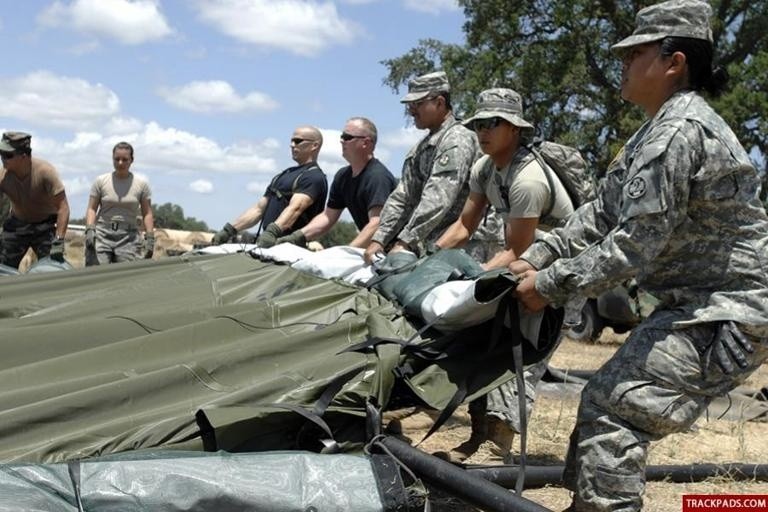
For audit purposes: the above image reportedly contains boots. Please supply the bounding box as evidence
[462,420,518,469]
[432,419,489,467]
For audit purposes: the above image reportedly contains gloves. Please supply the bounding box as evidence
[142,232,156,259]
[84,224,97,250]
[50,238,66,265]
[211,221,238,248]
[692,316,753,383]
[277,230,307,250]
[255,222,279,248]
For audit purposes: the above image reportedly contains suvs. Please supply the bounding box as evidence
[564,285,636,342]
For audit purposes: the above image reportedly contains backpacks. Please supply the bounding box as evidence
[480,141,593,227]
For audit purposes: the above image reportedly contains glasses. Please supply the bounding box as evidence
[405,92,444,112]
[289,138,316,144]
[340,133,368,143]
[473,116,507,131]
[0,152,25,160]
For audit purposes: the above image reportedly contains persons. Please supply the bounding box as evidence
[364,71,505,433]
[85,142,155,267]
[274,117,407,254]
[508,0,768,512]
[212,126,328,248]
[0,131,70,270]
[428,88,590,469]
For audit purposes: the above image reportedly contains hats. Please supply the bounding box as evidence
[0,133,31,150]
[611,0,718,52]
[460,88,536,134]
[399,70,452,100]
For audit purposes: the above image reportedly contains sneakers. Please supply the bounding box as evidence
[386,408,448,433]
[380,406,417,426]
